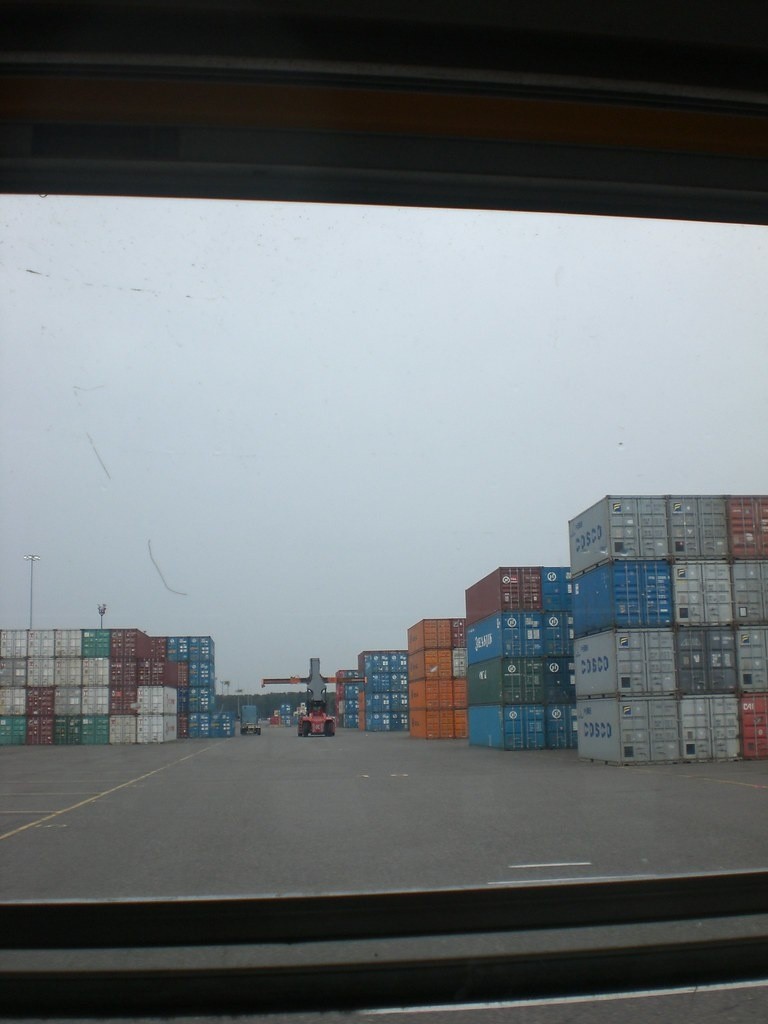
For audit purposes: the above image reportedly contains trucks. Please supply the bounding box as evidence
[240,705,262,735]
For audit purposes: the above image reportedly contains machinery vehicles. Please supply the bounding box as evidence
[263,658,368,737]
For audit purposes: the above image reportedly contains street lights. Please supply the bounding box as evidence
[23,555,40,628]
